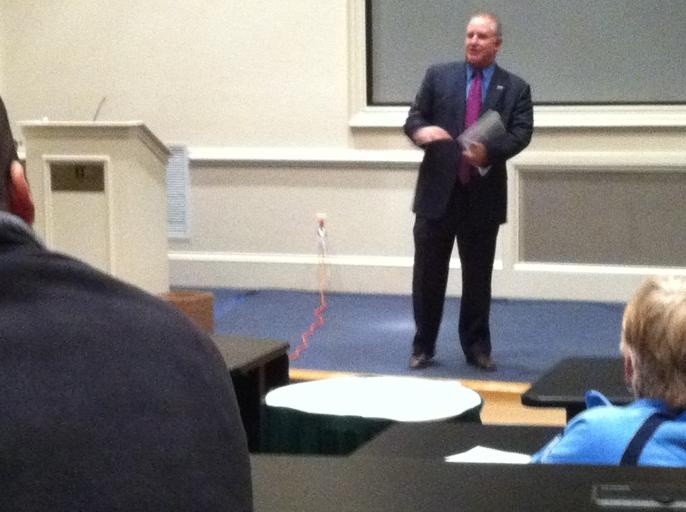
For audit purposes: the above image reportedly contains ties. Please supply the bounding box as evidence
[458,69,483,186]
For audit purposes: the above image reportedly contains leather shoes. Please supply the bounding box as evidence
[409,352,434,369]
[467,352,497,371]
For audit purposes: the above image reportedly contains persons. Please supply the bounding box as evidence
[527,272,686,470]
[402,11,533,371]
[0,97,252,511]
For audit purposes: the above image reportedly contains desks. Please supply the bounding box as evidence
[344,421,565,463]
[204,336,291,454]
[259,374,487,459]
[520,354,636,421]
